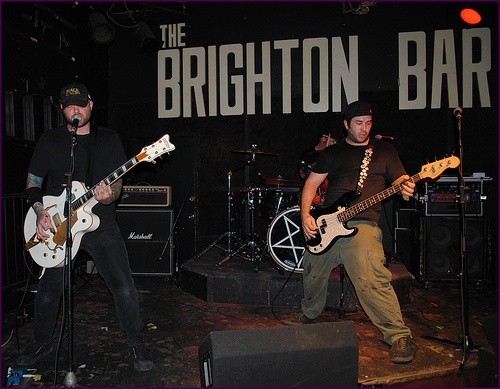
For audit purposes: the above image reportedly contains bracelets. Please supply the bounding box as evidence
[26,186,43,206]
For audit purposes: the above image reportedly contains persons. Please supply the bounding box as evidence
[303,127,343,162]
[281,100,417,362]
[16,82,154,372]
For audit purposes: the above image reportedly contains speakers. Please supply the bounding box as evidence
[116,208,174,276]
[197,320,360,388]
[417,217,490,283]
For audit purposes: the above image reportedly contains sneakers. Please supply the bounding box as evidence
[391,338,414,363]
[282,313,312,325]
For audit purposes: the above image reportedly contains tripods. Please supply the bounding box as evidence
[419,118,484,377]
[191,155,287,275]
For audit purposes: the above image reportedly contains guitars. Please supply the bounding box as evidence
[23,133,177,269]
[302,153,462,256]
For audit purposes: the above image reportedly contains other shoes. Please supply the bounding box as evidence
[130,345,154,372]
[16,338,51,366]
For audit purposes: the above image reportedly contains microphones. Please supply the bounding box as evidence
[71,114,82,132]
[453,107,462,118]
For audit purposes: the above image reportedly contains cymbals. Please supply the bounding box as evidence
[229,148,277,157]
[264,178,303,187]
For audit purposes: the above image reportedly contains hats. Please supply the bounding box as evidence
[60,82,91,107]
[344,101,373,120]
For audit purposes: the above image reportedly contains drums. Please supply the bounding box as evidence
[231,187,267,209]
[266,204,315,274]
[274,190,301,213]
